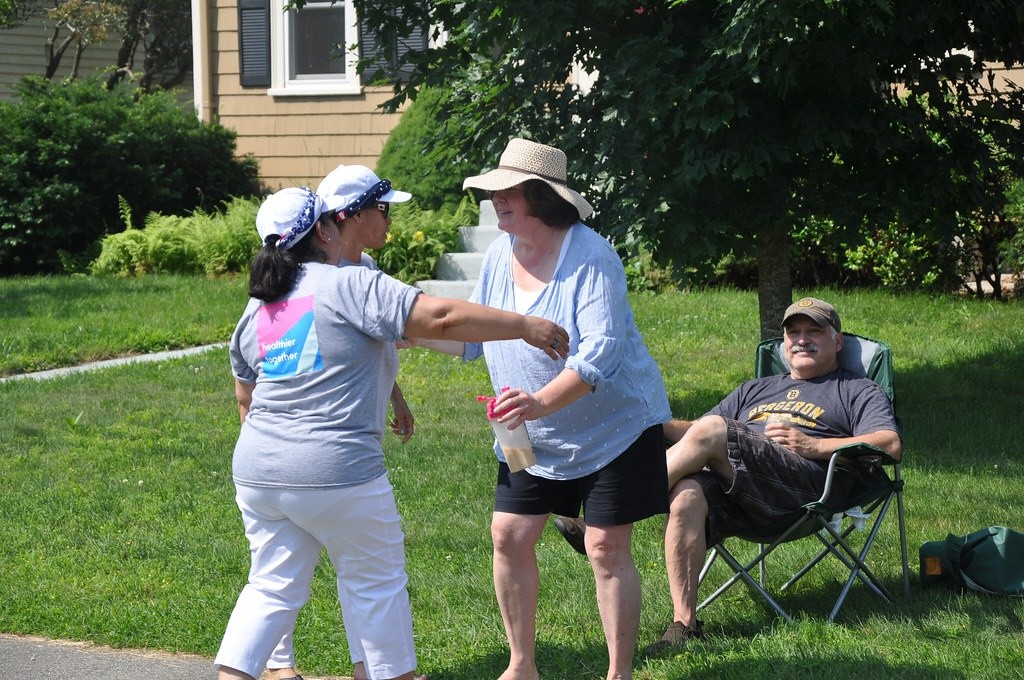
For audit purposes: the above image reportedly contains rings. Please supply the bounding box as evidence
[785,437,788,443]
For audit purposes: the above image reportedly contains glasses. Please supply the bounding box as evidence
[366,202,390,219]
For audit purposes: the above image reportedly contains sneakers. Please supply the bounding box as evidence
[552,514,588,557]
[641,620,708,658]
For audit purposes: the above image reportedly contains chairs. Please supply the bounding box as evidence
[695,332,912,626]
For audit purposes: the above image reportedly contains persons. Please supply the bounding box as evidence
[554,298,903,657]
[410,137,668,680]
[210,187,572,680]
[216,163,421,680]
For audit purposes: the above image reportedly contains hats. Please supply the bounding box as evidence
[256,186,346,250]
[461,136,595,220]
[778,297,842,334]
[316,165,413,223]
